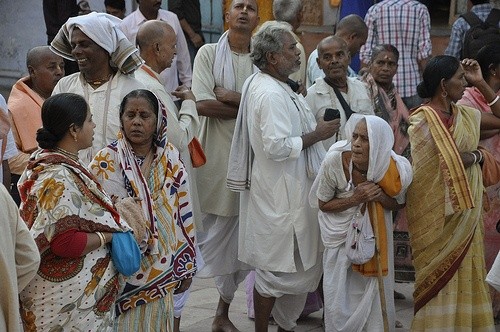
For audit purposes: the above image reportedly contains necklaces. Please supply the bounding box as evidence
[84,72,111,85]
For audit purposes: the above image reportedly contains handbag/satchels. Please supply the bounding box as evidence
[187,137,207,168]
[344,201,375,264]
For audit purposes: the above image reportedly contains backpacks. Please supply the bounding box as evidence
[460,7,500,81]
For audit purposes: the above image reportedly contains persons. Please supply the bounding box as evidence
[50,11,147,170]
[87,89,198,332]
[263,0,306,86]
[121,0,192,103]
[306,14,369,89]
[225,21,341,332]
[360,0,500,332]
[104,0,125,19]
[300,35,376,317]
[134,20,204,332]
[360,45,416,299]
[308,114,414,332]
[18,93,132,332]
[173,0,262,332]
[173,0,205,59]
[0,183,40,332]
[7,46,65,206]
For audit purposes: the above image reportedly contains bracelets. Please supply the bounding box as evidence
[487,96,499,106]
[472,150,482,163]
[96,231,106,247]
